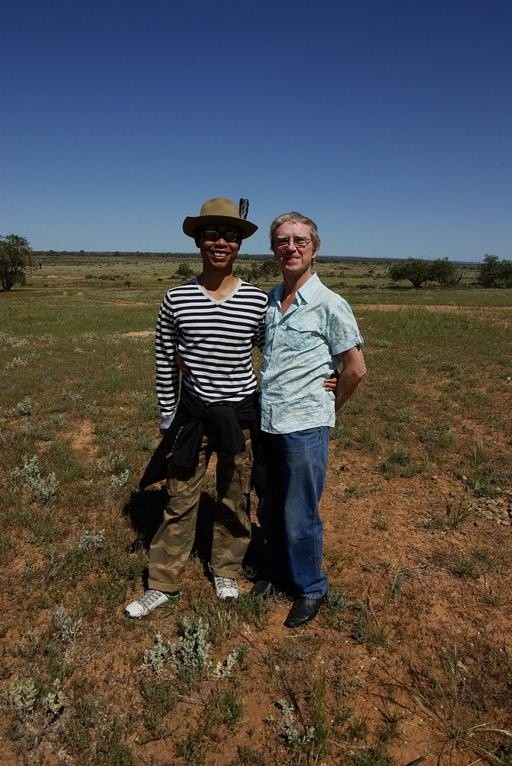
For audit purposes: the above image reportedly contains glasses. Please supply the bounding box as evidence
[199,228,242,242]
[276,236,312,247]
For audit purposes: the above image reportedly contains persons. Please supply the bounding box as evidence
[173,212,368,629]
[121,198,339,621]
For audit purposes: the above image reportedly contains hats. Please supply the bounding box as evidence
[182,198,258,239]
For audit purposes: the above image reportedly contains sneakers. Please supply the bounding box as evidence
[124,589,183,620]
[213,576,239,602]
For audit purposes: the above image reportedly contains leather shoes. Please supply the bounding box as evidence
[249,576,275,602]
[283,588,329,628]
